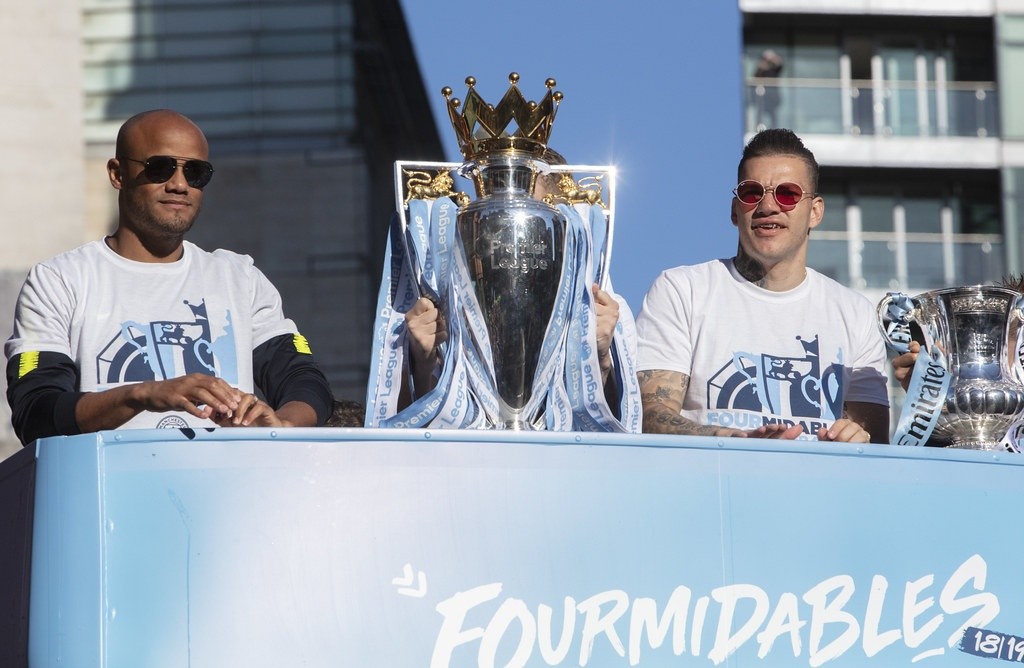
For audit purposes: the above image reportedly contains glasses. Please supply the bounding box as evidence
[118,155,216,188]
[732,179,818,206]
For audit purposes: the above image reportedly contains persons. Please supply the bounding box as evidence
[4,107,336,446]
[891,340,920,394]
[404,145,620,406]
[636,128,887,443]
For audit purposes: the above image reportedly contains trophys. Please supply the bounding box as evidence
[878,286,1023,451]
[393,73,619,431]
